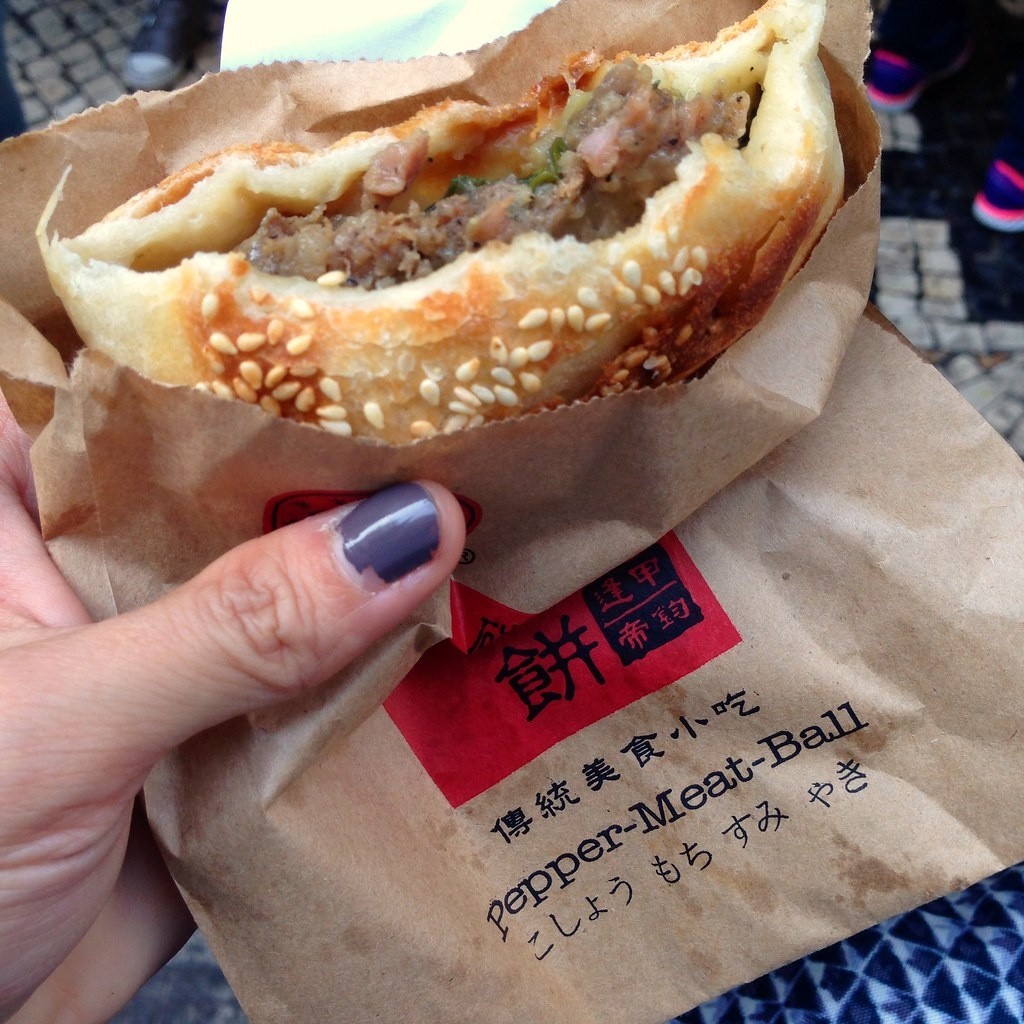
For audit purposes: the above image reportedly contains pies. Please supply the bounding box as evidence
[31,0,849,438]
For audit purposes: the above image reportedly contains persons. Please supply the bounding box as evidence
[862,0,1024,233]
[0,394,463,1024]
[121,0,207,93]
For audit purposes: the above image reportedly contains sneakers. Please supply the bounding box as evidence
[972,160,1024,232]
[863,48,919,113]
[122,0,209,91]
